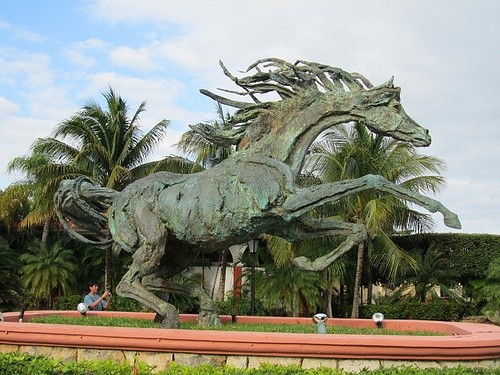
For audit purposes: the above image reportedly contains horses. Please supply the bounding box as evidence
[50,56,463,329]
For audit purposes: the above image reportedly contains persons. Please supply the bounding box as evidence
[84,279,113,311]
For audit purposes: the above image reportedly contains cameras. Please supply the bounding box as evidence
[105,292,112,298]
